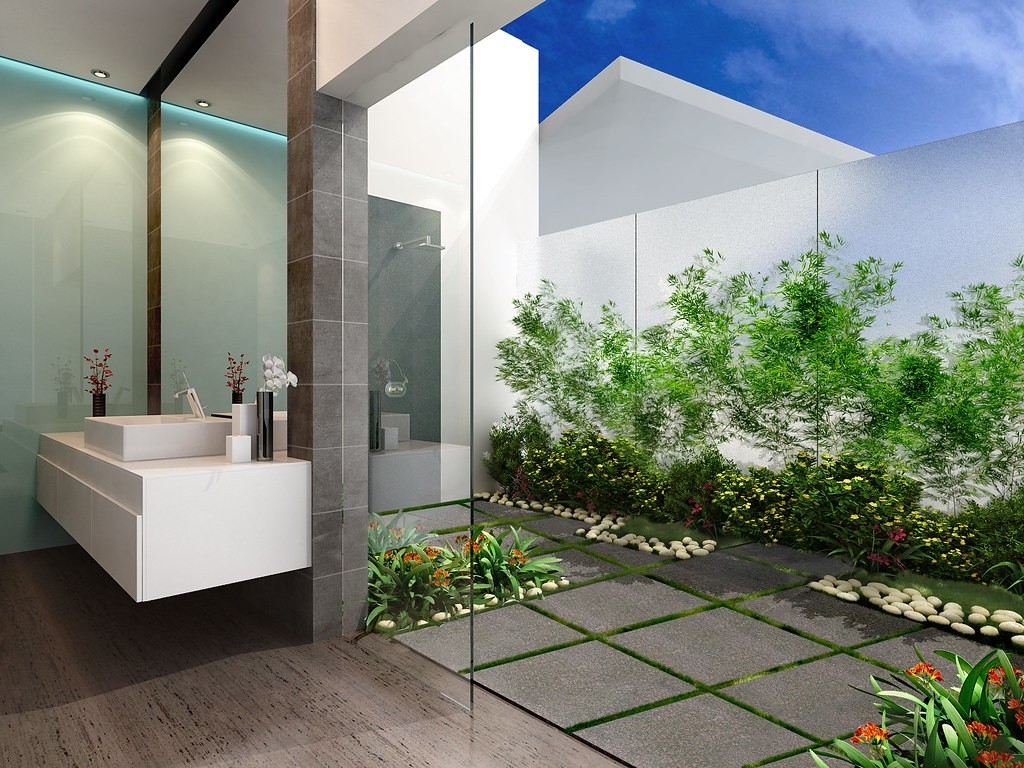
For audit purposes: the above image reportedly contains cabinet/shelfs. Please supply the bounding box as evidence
[35,452,146,603]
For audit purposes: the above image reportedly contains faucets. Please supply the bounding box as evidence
[174,388,209,417]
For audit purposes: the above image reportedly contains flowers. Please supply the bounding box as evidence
[50,356,74,393]
[368,356,392,392]
[169,358,188,397]
[224,352,250,394]
[84,347,113,394]
[256,354,298,398]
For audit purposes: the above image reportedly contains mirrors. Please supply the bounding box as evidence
[159,0,289,418]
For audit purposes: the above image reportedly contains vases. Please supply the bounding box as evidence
[231,393,243,404]
[175,398,183,414]
[92,393,106,417]
[370,389,380,453]
[58,392,69,419]
[257,392,274,463]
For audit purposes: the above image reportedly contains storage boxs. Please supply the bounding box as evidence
[380,426,400,451]
[226,435,251,463]
[232,403,257,459]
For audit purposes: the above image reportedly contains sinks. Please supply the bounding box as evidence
[380,412,411,441]
[83,413,232,463]
